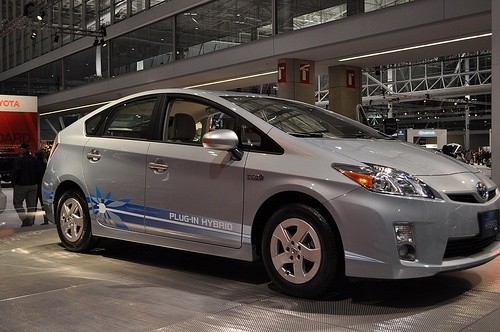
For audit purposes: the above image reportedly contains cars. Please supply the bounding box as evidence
[41,89,500,300]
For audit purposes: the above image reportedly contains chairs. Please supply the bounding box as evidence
[170,113,196,143]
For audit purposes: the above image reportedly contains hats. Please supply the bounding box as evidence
[19,144,29,150]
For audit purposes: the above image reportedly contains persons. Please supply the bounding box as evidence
[33,149,50,226]
[458,147,493,167]
[9,143,43,229]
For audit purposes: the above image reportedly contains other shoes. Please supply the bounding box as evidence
[28,224,33,226]
[40,222,49,226]
[21,218,31,227]
